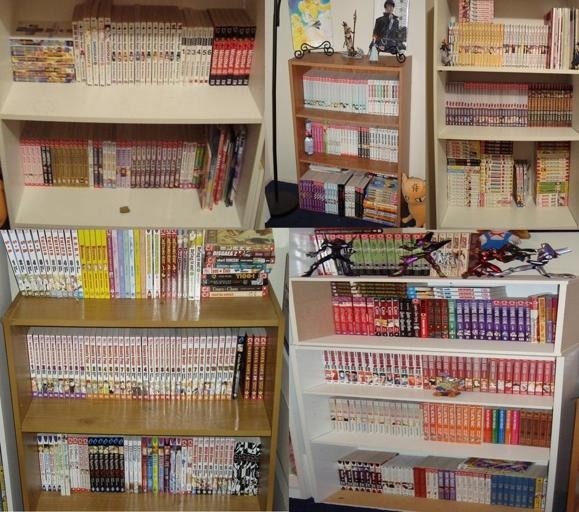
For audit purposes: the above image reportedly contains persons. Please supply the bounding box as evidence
[373,0,400,41]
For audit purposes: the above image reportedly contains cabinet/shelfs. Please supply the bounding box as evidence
[0,0,579,512]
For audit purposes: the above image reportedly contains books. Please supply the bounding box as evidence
[8,0,257,88]
[302,68,400,117]
[323,350,556,397]
[446,139,571,210]
[304,120,399,163]
[448,0,579,70]
[336,449,547,510]
[298,165,399,227]
[332,396,553,448]
[444,82,573,127]
[312,230,470,277]
[26,326,267,401]
[331,282,558,345]
[0,228,275,301]
[18,125,247,212]
[36,432,271,496]
